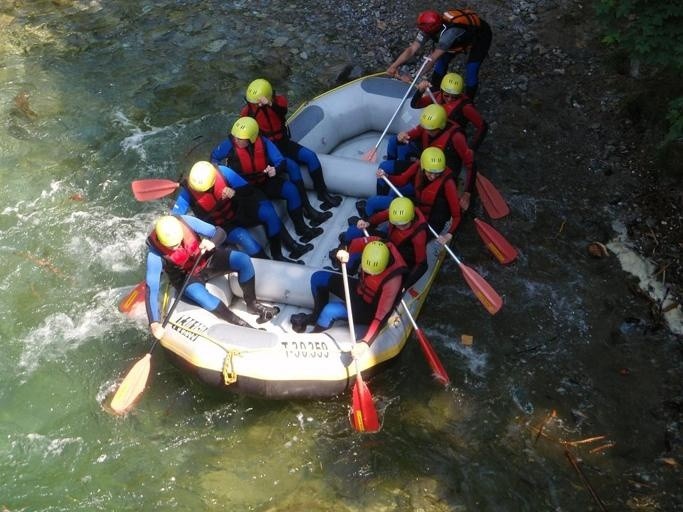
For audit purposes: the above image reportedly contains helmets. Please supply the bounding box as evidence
[420,146,447,173]
[420,103,447,131]
[388,197,417,226]
[244,77,273,106]
[229,116,260,146]
[155,215,185,249]
[361,241,391,276]
[416,9,443,35]
[440,72,465,95]
[188,160,217,193]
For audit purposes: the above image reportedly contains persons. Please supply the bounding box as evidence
[385,8,492,100]
[145,213,280,340]
[386,72,487,162]
[329,197,429,289]
[347,148,461,247]
[290,236,408,361]
[169,160,304,265]
[377,103,474,211]
[239,79,342,228]
[211,117,323,260]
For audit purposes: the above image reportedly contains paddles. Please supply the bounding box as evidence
[118,279,147,314]
[112,247,207,412]
[382,174,503,315]
[364,229,450,385]
[362,56,431,163]
[427,87,510,219]
[132,180,180,202]
[342,263,380,432]
[408,139,518,266]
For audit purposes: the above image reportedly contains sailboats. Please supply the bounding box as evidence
[142,69,477,399]
